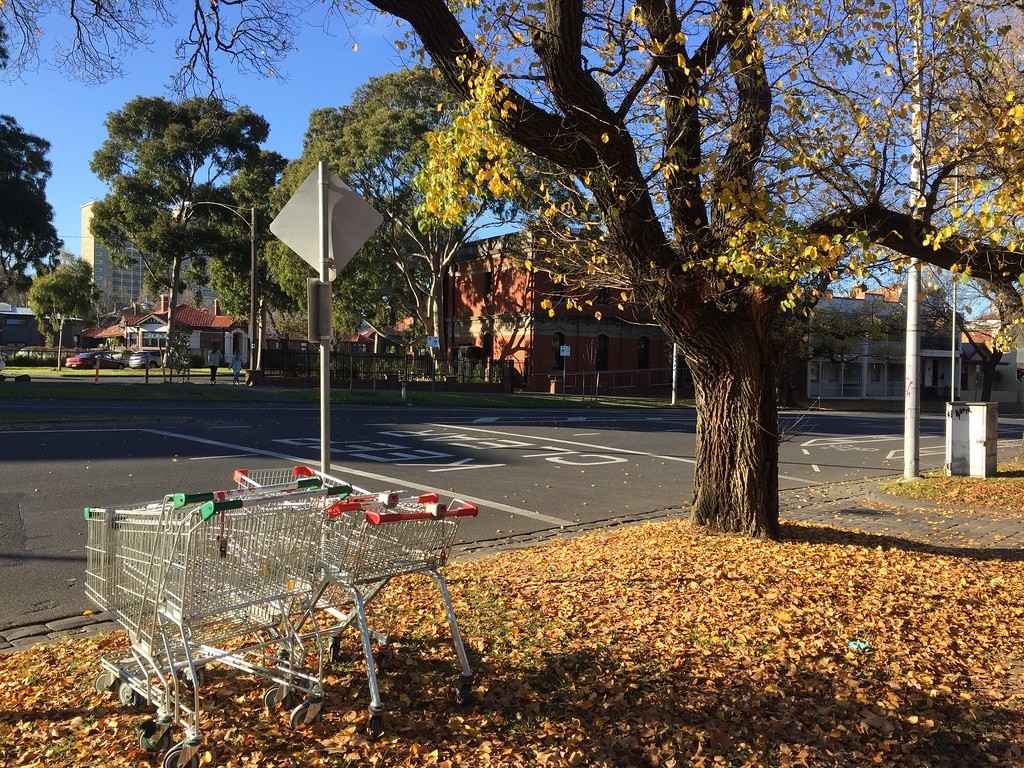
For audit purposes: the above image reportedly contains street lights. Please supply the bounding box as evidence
[182,202,266,388]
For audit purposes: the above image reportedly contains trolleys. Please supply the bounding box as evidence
[82,465,481,768]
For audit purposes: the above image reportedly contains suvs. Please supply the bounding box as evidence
[129,351,164,368]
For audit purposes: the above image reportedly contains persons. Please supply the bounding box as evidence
[208,344,223,385]
[228,348,244,385]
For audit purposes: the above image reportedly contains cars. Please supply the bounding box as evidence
[65,352,127,370]
